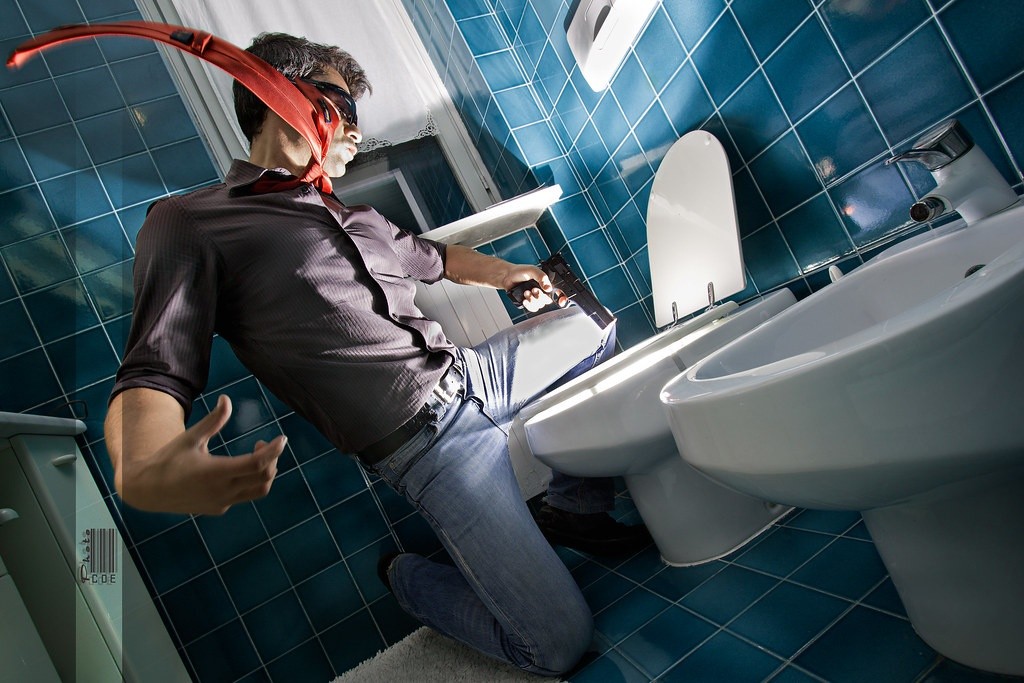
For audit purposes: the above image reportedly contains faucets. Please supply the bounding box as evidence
[881,121,1019,226]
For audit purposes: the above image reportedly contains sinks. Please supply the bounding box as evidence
[661,199,1024,675]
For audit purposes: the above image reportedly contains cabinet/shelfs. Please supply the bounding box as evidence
[0,433,193,683]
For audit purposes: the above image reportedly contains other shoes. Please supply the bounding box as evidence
[555,513,648,550]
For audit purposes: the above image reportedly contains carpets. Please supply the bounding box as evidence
[329,626,568,683]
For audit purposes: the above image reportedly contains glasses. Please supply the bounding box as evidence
[290,78,357,127]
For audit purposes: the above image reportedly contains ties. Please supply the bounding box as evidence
[8,21,342,193]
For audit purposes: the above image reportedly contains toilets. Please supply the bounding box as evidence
[521,124,798,573]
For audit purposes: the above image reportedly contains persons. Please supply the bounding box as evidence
[106,33,616,674]
